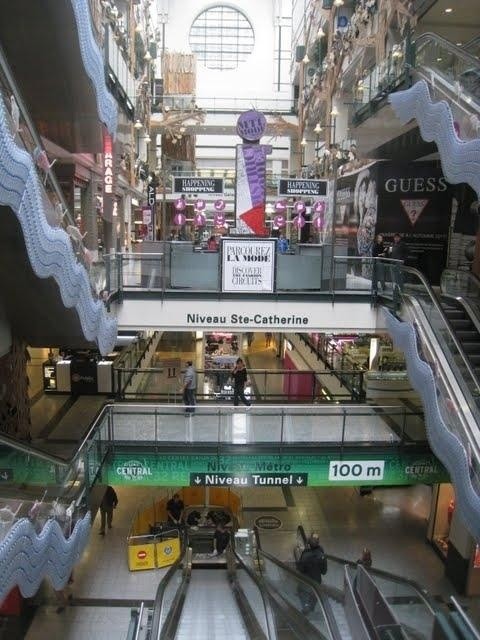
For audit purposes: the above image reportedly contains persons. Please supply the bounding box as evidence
[306,235,316,244]
[230,356,252,411]
[278,235,289,255]
[207,235,217,249]
[372,233,387,290]
[179,358,197,416]
[265,332,273,348]
[351,548,374,589]
[212,522,230,556]
[295,532,328,615]
[49,571,73,614]
[166,494,185,527]
[246,333,253,346]
[390,232,410,290]
[98,485,118,536]
